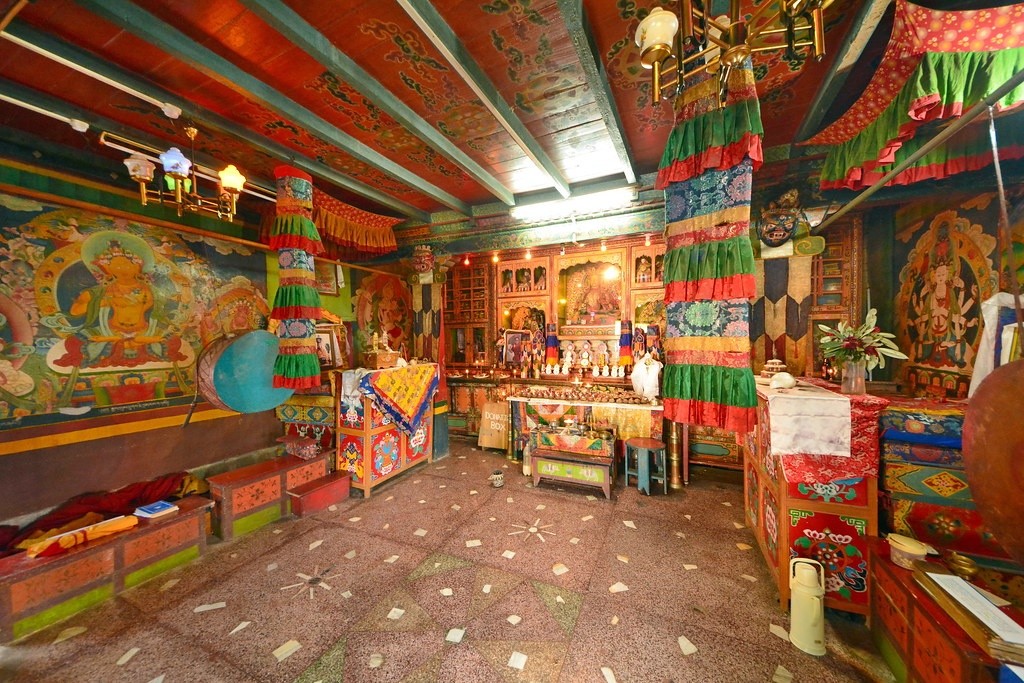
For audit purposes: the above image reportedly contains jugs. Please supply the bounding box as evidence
[789,556,827,656]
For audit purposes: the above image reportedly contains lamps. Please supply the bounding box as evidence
[123,128,246,223]
[635,0,835,110]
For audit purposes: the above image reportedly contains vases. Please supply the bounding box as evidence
[840,360,866,395]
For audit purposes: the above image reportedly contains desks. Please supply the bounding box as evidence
[506,385,664,486]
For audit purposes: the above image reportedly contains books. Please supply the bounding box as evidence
[133,500,179,519]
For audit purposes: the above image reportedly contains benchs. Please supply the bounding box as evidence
[0,494,215,647]
[205,446,338,542]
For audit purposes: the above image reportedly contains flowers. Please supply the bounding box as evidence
[817,308,909,373]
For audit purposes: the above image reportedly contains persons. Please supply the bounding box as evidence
[636,257,652,283]
[572,266,619,315]
[501,272,513,292]
[534,269,546,290]
[509,337,522,361]
[516,270,531,292]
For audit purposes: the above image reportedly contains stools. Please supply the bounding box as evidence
[287,469,352,516]
[624,437,667,495]
